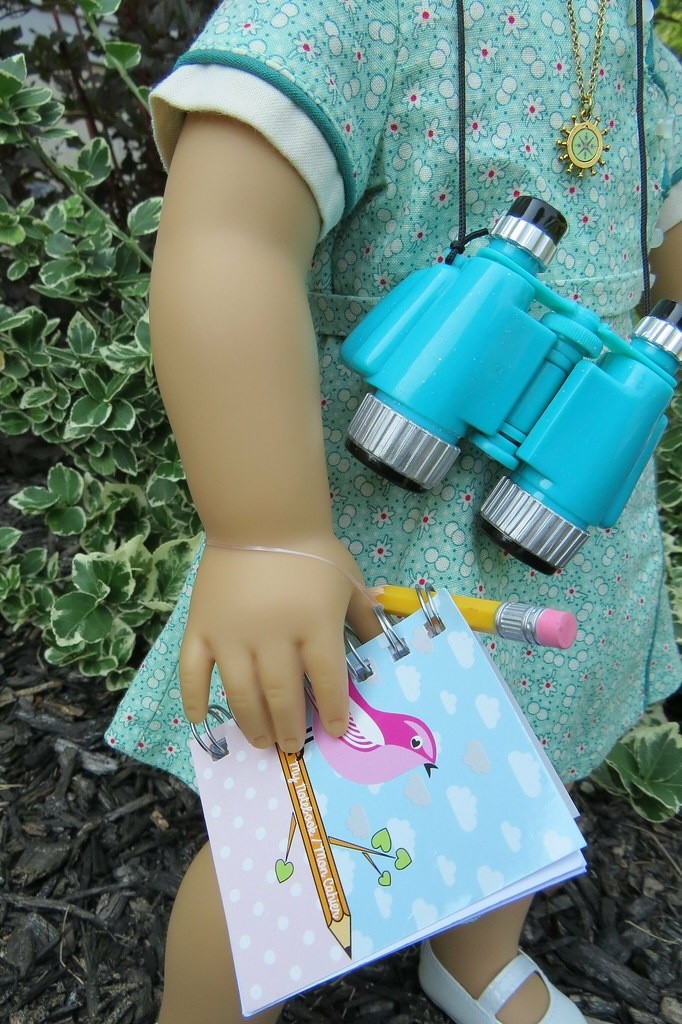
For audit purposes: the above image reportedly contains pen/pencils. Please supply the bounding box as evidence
[368,585,577,649]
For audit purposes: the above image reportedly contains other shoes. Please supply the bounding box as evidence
[419,937,590,1024]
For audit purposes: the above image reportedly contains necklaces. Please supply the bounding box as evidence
[555,0,611,179]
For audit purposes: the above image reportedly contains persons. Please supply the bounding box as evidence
[103,0,682,1024]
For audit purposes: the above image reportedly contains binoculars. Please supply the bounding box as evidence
[340,195,682,575]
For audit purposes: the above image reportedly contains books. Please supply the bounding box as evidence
[183,582,589,1016]
